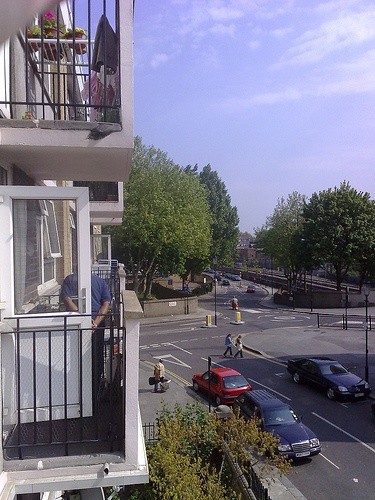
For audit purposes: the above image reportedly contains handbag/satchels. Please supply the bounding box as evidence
[149,376,156,385]
[160,378,169,392]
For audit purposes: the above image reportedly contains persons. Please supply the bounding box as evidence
[233,336,243,359]
[230,296,238,307]
[62,263,111,404]
[93,253,104,265]
[223,333,233,357]
[152,359,165,392]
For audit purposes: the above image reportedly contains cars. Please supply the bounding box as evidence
[204,268,242,281]
[247,285,256,293]
[286,356,371,403]
[222,280,230,286]
[192,368,252,408]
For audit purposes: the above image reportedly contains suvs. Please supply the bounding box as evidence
[231,389,321,462]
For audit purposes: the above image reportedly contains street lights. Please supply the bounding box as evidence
[362,283,370,383]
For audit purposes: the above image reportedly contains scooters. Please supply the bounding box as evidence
[230,301,239,310]
